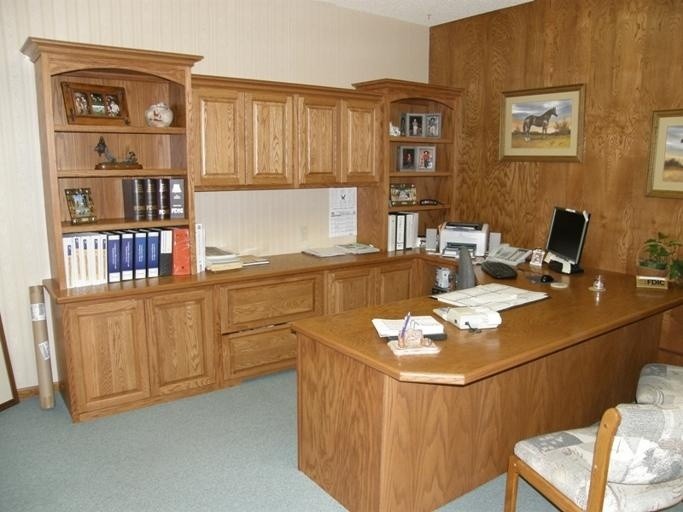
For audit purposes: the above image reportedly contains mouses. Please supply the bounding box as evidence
[541,275,553,284]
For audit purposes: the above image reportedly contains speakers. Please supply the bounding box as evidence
[425,229,437,251]
[489,233,501,252]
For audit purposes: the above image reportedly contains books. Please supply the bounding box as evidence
[431,283,550,313]
[124,177,184,219]
[64,227,191,287]
[194,225,269,272]
[388,211,418,251]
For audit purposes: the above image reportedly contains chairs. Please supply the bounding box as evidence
[502,402,683,512]
[636,359,683,410]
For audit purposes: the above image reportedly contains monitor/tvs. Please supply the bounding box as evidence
[543,206,591,275]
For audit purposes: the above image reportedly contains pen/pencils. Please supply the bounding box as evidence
[401,311,410,337]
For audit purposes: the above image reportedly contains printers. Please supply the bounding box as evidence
[439,222,489,258]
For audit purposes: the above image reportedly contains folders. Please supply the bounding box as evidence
[387,214,396,252]
[396,215,406,251]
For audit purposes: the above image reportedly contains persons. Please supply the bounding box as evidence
[406,152,411,168]
[412,117,418,135]
[428,117,436,135]
[422,152,429,168]
[73,91,119,114]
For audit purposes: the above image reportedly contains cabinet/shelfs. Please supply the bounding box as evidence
[191,76,295,193]
[19,36,203,290]
[353,79,466,253]
[42,244,420,424]
[293,84,383,188]
[422,248,473,298]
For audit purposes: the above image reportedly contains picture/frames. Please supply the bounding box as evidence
[396,145,416,171]
[416,145,436,170]
[389,185,417,206]
[62,81,130,126]
[425,113,442,139]
[406,114,425,138]
[645,108,683,199]
[399,112,406,135]
[64,187,99,223]
[500,84,585,162]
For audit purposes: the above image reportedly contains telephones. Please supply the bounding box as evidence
[486,243,532,266]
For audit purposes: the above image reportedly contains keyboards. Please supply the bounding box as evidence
[481,260,517,279]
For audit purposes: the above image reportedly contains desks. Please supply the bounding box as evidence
[288,254,683,512]
